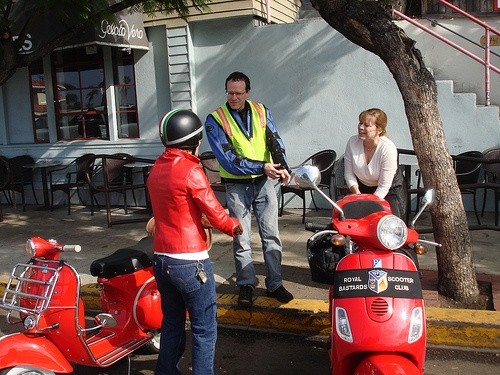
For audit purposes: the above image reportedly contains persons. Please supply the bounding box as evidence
[203,70,295,303]
[144,109,242,375]
[342,107,409,260]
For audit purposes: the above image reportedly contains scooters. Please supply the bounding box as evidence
[0,220,214,375]
[295,165,443,375]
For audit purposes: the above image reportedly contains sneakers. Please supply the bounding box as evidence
[238,284,252,306]
[267,285,293,302]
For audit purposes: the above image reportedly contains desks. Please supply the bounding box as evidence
[398,154,429,231]
[121,158,157,218]
[21,158,67,214]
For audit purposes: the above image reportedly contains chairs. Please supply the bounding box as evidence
[0,147,500,236]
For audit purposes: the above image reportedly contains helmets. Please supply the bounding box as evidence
[159,109,203,150]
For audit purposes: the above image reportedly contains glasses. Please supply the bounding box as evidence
[225,90,247,98]
